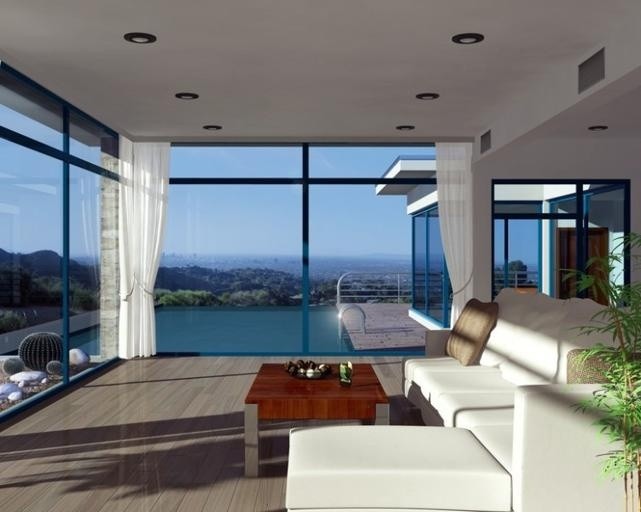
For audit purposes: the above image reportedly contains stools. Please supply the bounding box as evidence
[281,424,512,511]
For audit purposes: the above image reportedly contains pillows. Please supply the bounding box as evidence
[442,297,501,368]
[564,344,641,387]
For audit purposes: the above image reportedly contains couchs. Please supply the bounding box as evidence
[397,284,641,511]
